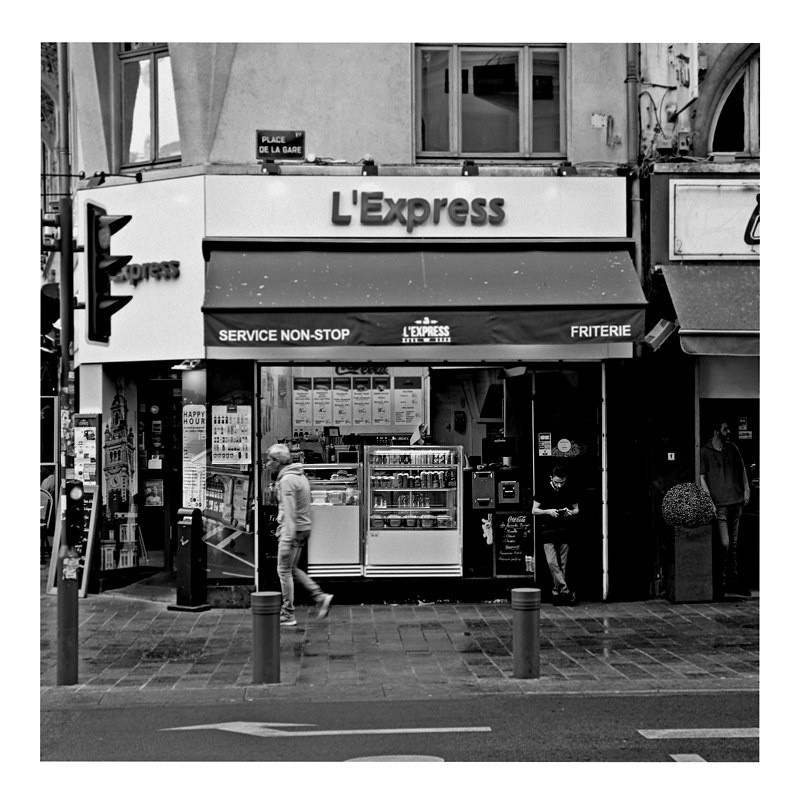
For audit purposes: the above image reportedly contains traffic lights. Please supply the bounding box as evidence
[84,200,133,346]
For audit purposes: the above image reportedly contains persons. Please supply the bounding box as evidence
[146,487,162,506]
[266,444,334,626]
[532,465,580,607]
[700,421,751,587]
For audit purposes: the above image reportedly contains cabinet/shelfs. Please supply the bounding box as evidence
[302,465,362,507]
[368,447,458,528]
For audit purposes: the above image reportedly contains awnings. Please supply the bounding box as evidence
[650,265,759,356]
[201,246,647,361]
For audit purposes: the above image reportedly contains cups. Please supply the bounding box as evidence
[503,457,512,466]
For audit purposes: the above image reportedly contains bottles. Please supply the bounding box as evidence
[214,415,248,459]
[371,453,456,489]
[328,442,335,463]
[295,428,319,441]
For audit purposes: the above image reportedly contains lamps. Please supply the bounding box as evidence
[558,162,578,177]
[170,360,193,371]
[462,159,479,176]
[362,161,377,176]
[87,162,143,186]
[260,162,280,175]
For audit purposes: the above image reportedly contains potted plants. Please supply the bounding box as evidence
[662,482,714,604]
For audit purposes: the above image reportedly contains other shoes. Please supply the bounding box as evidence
[566,591,577,607]
[313,593,334,621]
[725,580,752,596]
[279,608,297,626]
[552,594,562,607]
